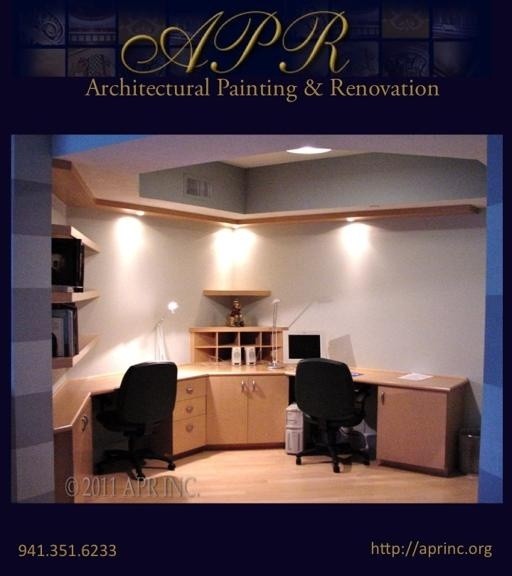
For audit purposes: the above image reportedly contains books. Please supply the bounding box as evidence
[52,238,85,357]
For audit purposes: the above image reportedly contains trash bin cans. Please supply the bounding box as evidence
[457,427,481,474]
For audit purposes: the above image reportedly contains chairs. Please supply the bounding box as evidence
[293,356,375,474]
[94,362,177,482]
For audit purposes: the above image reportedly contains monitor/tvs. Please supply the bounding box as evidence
[283,330,323,364]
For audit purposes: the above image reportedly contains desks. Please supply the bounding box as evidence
[51,364,469,503]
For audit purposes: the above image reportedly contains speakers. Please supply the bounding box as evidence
[231,347,242,366]
[245,347,256,366]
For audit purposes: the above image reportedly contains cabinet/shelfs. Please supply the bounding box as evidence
[189,326,288,366]
[51,223,99,369]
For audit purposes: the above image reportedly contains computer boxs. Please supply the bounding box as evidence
[285,401,309,454]
